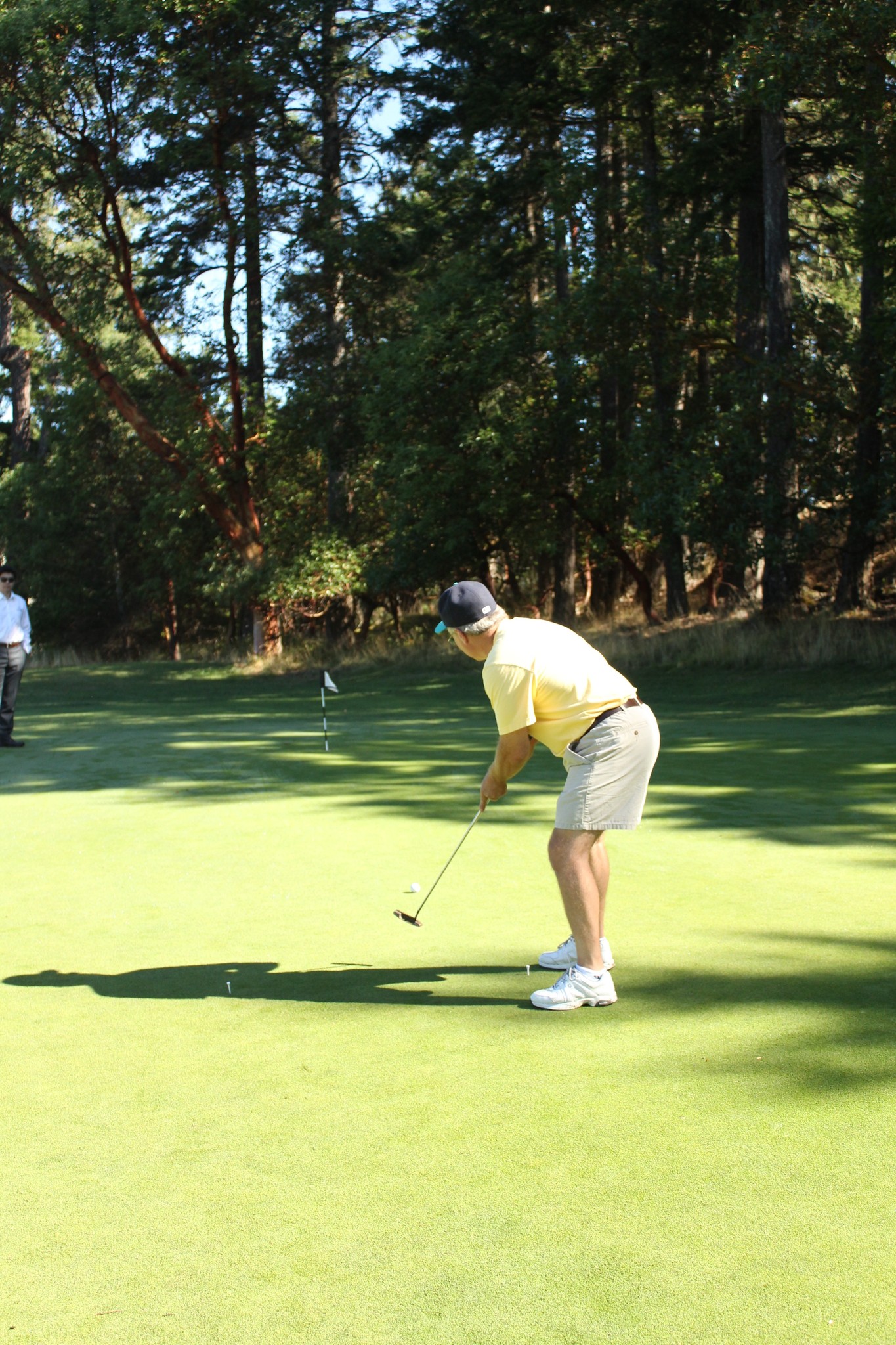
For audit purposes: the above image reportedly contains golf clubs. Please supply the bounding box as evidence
[392,796,490,927]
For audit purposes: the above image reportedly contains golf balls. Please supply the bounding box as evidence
[410,881,421,893]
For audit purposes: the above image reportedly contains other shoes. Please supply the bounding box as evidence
[0,736,24,747]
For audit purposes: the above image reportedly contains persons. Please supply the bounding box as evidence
[434,580,660,1011]
[0,565,34,748]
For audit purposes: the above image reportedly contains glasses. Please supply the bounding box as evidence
[0,577,15,582]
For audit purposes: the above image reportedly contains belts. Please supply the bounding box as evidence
[0,642,20,649]
[582,695,642,735]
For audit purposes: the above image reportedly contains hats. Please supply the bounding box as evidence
[433,581,497,635]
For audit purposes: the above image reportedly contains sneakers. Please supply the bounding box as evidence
[530,960,617,1009]
[537,930,617,971]
